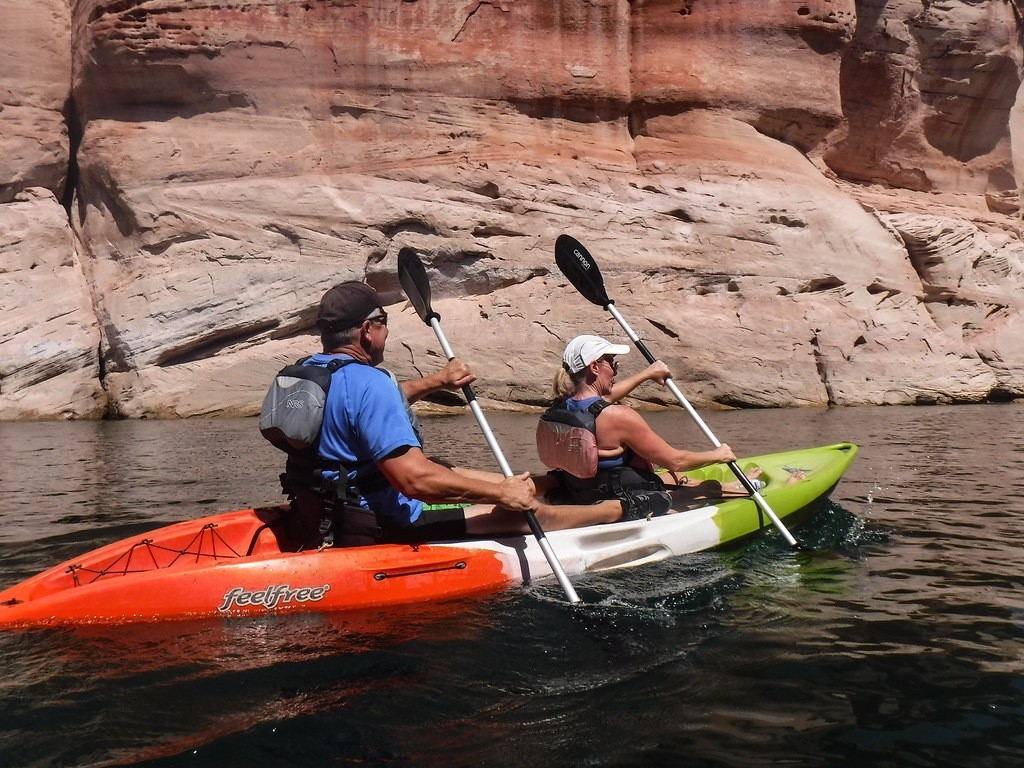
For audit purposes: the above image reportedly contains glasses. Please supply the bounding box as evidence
[597,359,620,371]
[375,317,387,327]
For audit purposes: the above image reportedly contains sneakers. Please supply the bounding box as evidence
[544,467,574,505]
[593,486,673,523]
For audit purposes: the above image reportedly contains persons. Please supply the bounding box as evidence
[536,335,805,515]
[278,279,673,542]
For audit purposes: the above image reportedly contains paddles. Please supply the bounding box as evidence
[395,244,583,605]
[552,233,806,551]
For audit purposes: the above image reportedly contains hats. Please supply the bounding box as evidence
[316,279,400,332]
[563,337,630,374]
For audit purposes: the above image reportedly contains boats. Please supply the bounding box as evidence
[0,443,858,631]
